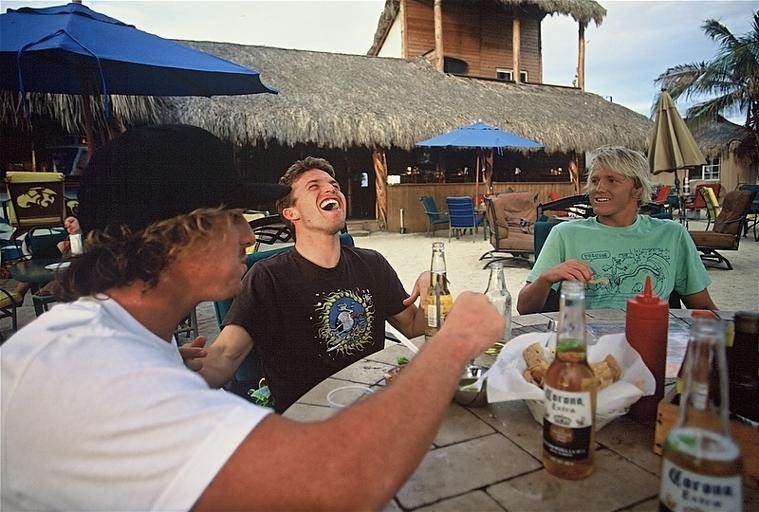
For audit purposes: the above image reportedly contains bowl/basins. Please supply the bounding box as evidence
[453,364,489,407]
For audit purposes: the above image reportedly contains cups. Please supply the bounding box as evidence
[326,386,374,415]
[68,234,83,253]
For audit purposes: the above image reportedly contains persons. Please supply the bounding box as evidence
[12,214,88,307]
[517,143,719,315]
[0,123,506,512]
[176,157,437,416]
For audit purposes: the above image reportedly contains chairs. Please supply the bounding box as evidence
[211,214,400,413]
[0,225,83,331]
[237,211,271,256]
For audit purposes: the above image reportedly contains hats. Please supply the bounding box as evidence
[74,121,294,239]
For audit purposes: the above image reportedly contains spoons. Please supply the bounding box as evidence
[459,368,490,394]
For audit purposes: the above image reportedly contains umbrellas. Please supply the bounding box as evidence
[0,0,279,163]
[647,87,708,213]
[414,119,545,208]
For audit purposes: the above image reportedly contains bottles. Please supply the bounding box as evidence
[424,241,453,340]
[656,317,744,512]
[626,275,670,424]
[541,280,598,481]
[653,309,715,458]
[483,263,511,355]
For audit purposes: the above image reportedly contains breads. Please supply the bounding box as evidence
[522,342,619,394]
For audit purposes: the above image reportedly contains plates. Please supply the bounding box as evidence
[44,262,74,268]
